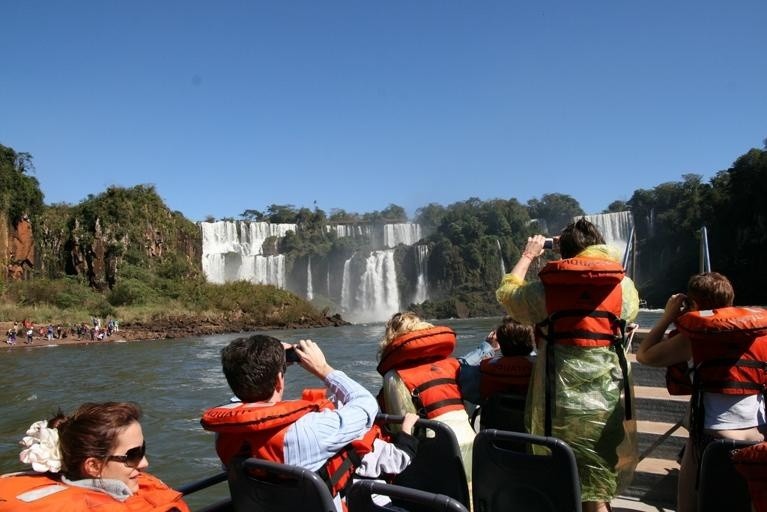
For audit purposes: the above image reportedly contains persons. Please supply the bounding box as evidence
[636,271,767,512]
[356,412,420,509]
[496,220,638,511]
[457,315,538,432]
[376,311,477,511]
[47,401,149,494]
[201,334,379,511]
[7,315,119,345]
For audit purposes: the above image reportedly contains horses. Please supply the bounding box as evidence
[286,347,301,362]
[543,238,553,249]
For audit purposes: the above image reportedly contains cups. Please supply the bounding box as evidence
[83,440,146,467]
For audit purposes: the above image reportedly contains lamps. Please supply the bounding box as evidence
[377,413,472,512]
[346,479,468,511]
[480,393,528,434]
[696,441,759,512]
[228,459,336,512]
[470,430,582,512]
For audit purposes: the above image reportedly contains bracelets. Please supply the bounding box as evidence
[522,252,533,261]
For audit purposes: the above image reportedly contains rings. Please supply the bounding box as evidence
[294,346,298,350]
